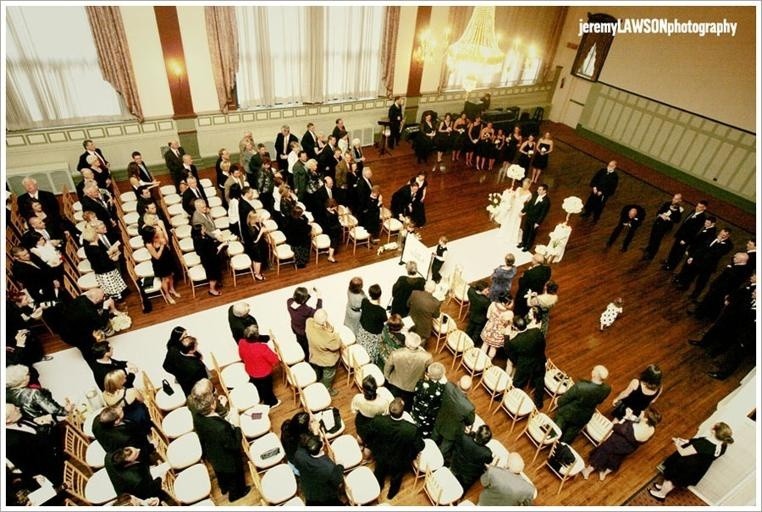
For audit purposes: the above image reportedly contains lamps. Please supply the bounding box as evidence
[412,7,541,92]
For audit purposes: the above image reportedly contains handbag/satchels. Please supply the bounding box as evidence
[162,379,174,396]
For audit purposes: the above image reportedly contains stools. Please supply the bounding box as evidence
[532,105,545,123]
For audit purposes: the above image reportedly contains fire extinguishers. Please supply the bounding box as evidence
[560,77,565,88]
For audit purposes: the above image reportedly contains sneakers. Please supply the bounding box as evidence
[270,400,281,409]
[361,457,373,466]
[329,388,338,396]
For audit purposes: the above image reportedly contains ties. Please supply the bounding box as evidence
[93,152,104,166]
[196,190,201,198]
[101,235,110,248]
[176,151,180,158]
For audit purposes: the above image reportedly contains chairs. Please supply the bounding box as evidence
[5,175,619,506]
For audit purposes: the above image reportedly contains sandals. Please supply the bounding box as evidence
[328,257,338,263]
[255,273,266,281]
[208,290,222,296]
[41,355,52,361]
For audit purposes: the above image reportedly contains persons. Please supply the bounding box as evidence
[7,95,755,505]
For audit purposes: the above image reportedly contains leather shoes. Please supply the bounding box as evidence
[416,157,538,185]
[689,339,727,381]
[517,242,524,248]
[370,240,378,245]
[639,247,673,272]
[364,244,373,249]
[671,275,707,328]
[646,487,666,502]
[580,213,629,254]
[229,486,251,502]
[653,481,662,491]
[523,249,529,251]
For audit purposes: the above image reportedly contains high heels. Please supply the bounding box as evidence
[167,296,176,304]
[169,290,181,298]
